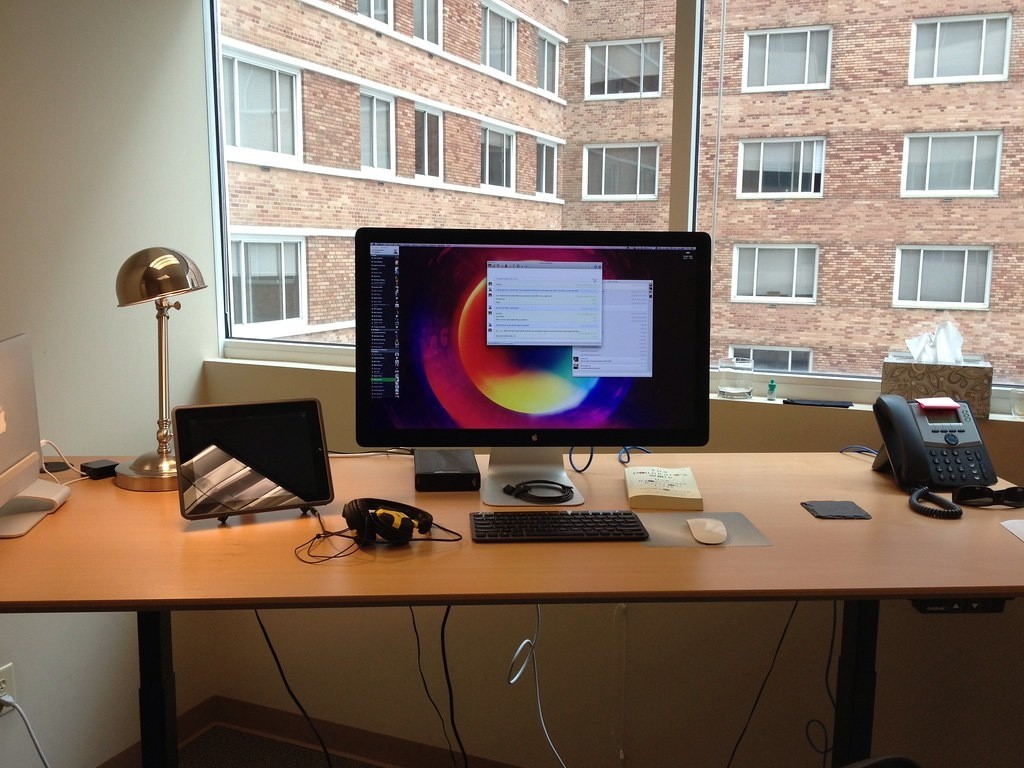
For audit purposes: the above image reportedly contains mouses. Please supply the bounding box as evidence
[686,518,728,545]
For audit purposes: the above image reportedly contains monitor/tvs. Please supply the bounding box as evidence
[354,227,710,508]
[0,334,70,539]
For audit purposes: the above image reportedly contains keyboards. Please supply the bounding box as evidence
[470,511,650,542]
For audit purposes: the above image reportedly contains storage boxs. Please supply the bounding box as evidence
[880,356,994,421]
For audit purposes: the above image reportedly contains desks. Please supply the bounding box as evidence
[0,451,1024,768]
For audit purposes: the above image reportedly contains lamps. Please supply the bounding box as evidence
[114,246,208,493]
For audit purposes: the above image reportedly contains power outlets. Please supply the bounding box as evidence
[0,662,18,716]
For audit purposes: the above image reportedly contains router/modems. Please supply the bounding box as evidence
[413,450,481,492]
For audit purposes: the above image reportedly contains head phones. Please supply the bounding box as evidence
[341,497,433,545]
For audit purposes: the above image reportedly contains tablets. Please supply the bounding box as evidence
[172,397,335,521]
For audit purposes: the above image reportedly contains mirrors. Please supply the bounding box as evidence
[171,396,335,524]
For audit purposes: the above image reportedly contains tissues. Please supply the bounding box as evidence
[880,321,994,419]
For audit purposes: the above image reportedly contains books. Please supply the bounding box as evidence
[624,467,703,511]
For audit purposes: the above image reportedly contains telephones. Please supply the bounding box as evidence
[872,394,998,491]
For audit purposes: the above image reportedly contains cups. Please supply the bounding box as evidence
[717,357,754,399]
[1008,389,1024,417]
